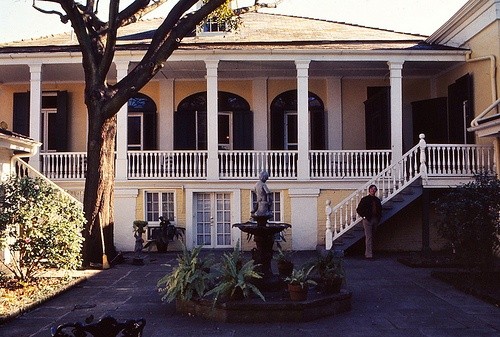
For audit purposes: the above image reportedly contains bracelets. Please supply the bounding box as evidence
[363,216,365,219]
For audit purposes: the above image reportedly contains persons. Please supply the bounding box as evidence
[250,170,274,215]
[355,184,384,259]
[0,121,16,136]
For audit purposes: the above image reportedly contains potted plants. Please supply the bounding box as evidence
[202,249,264,310]
[319,258,347,295]
[272,247,297,277]
[222,241,243,275]
[142,216,186,253]
[279,265,320,302]
[300,248,344,280]
[157,239,215,304]
